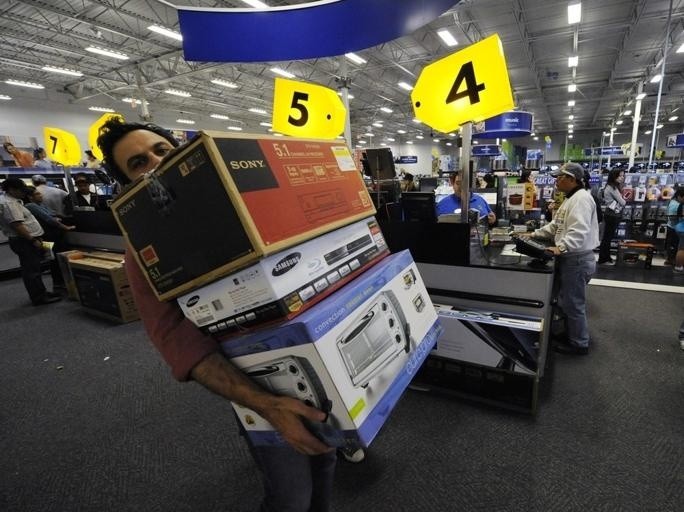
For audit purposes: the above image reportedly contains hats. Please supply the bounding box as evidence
[549,163,584,180]
[402,173,413,181]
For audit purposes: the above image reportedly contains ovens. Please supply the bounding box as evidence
[334,290,411,390]
[232,357,333,427]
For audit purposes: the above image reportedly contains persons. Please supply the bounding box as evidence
[671,202,684,275]
[516,167,535,185]
[597,166,627,266]
[337,441,367,465]
[97,118,340,511]
[1,136,103,308]
[544,170,603,224]
[398,167,413,181]
[663,189,684,265]
[512,161,600,354]
[433,170,497,226]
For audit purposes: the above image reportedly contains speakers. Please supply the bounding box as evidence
[366,148,396,179]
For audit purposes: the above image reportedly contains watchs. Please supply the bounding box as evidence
[557,245,569,255]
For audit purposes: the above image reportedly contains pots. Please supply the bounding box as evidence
[508,194,522,204]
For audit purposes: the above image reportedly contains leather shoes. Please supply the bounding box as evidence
[557,343,588,355]
[35,295,62,304]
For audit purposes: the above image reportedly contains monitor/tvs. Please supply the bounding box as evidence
[401,192,435,222]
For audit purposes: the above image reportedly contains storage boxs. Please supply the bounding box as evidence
[56,249,141,324]
[506,183,525,210]
[212,248,444,449]
[175,215,392,337]
[109,128,378,300]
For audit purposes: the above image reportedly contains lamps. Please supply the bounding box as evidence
[605,43,684,137]
[567,0,582,139]
[1,23,183,106]
[88,51,366,140]
[434,23,458,52]
[352,79,460,149]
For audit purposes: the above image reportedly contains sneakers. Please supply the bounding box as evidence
[337,437,365,463]
[599,259,615,266]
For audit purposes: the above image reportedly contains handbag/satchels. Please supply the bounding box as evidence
[605,208,623,224]
